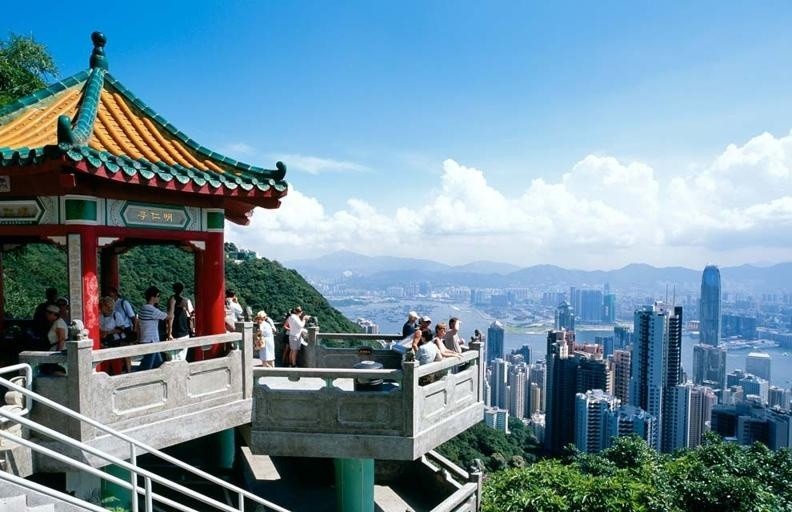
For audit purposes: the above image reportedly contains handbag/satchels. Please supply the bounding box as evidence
[392,334,415,354]
[255,339,264,349]
[103,331,125,345]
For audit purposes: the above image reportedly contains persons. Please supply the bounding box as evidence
[100,283,311,371]
[34,289,58,351]
[401,311,465,385]
[57,296,70,325]
[46,306,69,375]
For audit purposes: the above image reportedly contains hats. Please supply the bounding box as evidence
[46,305,59,312]
[409,311,417,318]
[421,317,431,322]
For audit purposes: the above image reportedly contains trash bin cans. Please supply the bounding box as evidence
[352,360,384,392]
